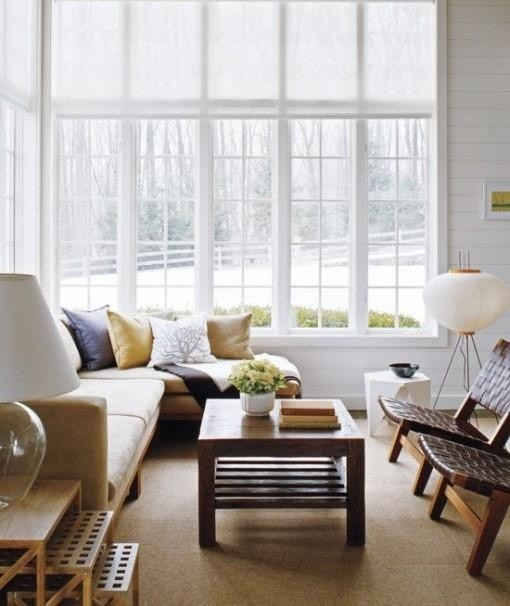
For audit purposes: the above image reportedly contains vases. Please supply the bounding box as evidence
[240,392,276,417]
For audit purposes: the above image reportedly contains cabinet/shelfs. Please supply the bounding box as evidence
[0,478,141,606]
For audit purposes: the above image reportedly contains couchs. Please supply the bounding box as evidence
[58,378,166,523]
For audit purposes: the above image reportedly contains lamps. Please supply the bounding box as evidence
[423,250,510,430]
[1,274,80,513]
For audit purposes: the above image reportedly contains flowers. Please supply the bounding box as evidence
[228,360,289,395]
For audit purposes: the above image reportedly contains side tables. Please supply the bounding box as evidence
[364,370,431,436]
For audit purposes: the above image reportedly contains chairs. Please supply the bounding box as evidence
[418,435,510,577]
[378,338,509,495]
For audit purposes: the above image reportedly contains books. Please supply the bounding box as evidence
[278,399,342,428]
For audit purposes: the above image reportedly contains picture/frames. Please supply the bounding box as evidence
[482,181,510,222]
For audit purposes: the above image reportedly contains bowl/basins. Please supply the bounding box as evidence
[390,362,420,378]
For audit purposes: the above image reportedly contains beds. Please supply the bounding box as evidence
[78,353,301,422]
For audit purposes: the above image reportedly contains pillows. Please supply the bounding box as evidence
[57,305,254,370]
